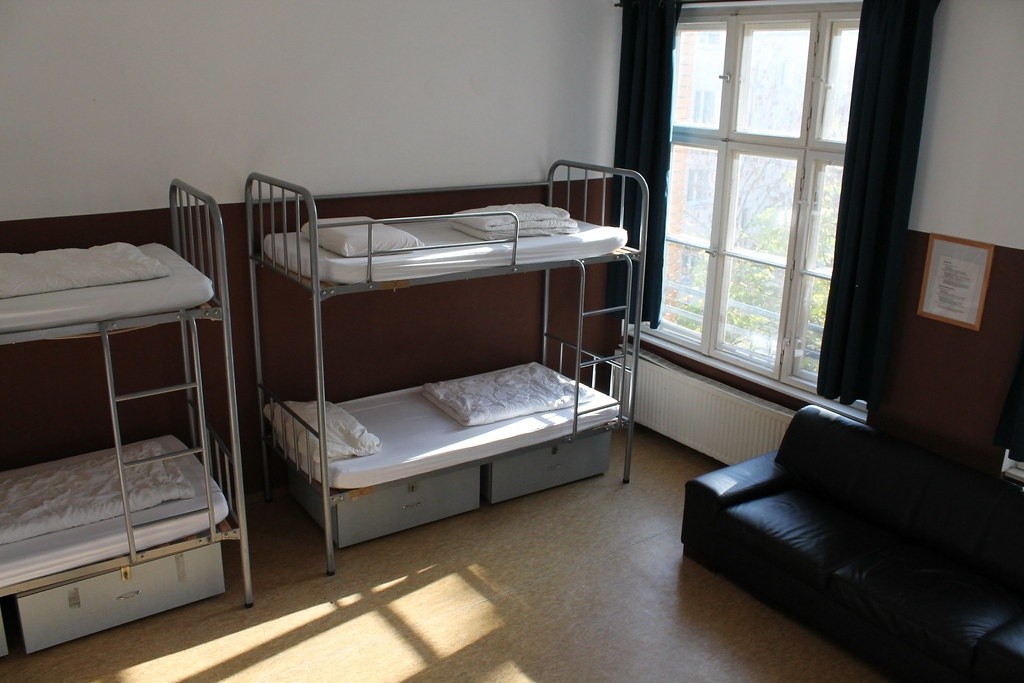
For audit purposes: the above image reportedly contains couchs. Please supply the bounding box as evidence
[682,404,1023,682]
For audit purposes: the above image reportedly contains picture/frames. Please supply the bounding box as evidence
[916,234,995,330]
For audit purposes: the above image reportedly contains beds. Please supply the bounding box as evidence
[0,436,233,595]
[263,204,640,298]
[0,244,214,345]
[273,363,627,507]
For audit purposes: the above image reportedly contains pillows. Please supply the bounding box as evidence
[300,216,424,258]
[264,401,382,464]
